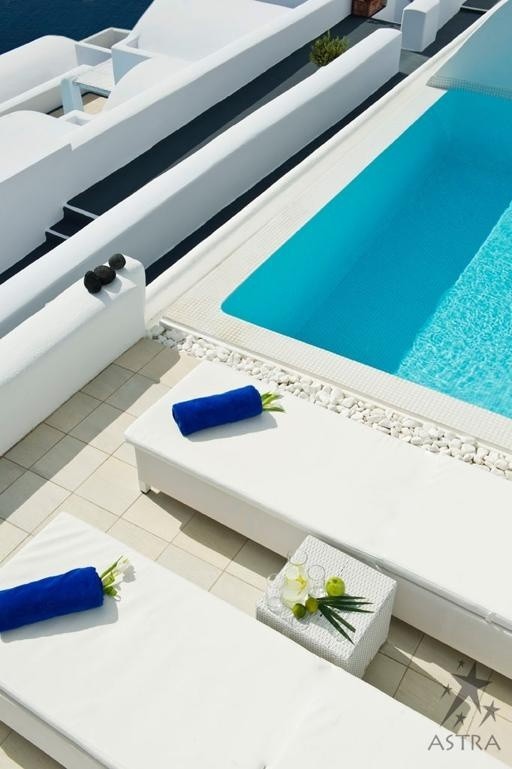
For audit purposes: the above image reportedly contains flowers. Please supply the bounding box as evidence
[98,554,137,603]
[260,381,285,417]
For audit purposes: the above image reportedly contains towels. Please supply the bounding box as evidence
[0,565,105,632]
[171,383,264,436]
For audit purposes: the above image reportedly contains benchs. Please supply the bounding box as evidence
[123,357,511,681]
[1,507,510,768]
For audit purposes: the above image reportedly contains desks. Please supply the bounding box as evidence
[253,533,398,680]
